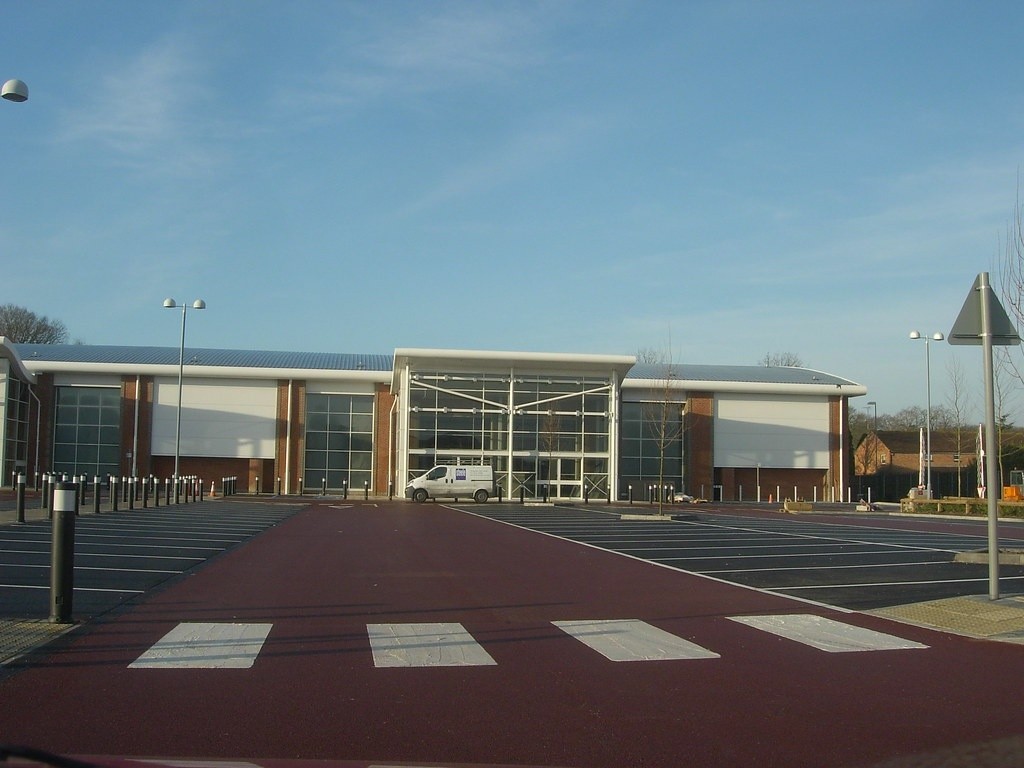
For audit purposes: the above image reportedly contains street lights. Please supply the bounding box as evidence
[867,401,879,502]
[163,297,206,495]
[909,331,944,500]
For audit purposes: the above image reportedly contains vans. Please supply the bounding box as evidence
[404,464,493,504]
